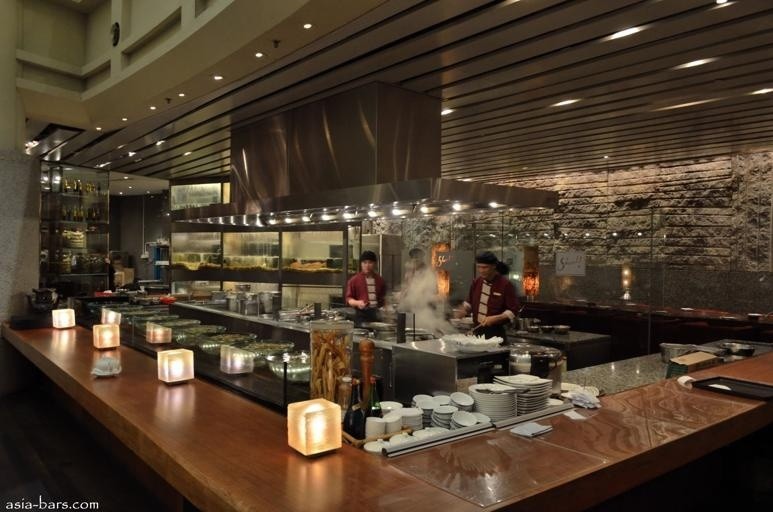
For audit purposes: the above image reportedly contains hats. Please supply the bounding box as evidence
[409,249,425,258]
[475,251,498,264]
[360,250,378,261]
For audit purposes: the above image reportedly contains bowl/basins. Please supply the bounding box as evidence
[528,325,572,335]
[364,391,491,453]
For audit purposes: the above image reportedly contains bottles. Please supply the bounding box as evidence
[63,178,102,221]
[309,312,383,440]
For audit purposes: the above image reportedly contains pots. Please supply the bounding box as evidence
[515,317,531,331]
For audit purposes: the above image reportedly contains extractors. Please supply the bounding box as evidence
[171,82,559,231]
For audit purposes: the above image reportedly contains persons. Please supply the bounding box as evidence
[345,251,386,329]
[394,248,438,309]
[454,251,517,339]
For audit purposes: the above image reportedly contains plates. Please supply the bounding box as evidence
[468,372,554,422]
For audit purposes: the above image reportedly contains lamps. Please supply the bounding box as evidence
[219,344,254,375]
[100,307,121,325]
[51,308,76,329]
[157,348,194,384]
[93,323,120,349]
[286,398,343,457]
[146,321,173,345]
